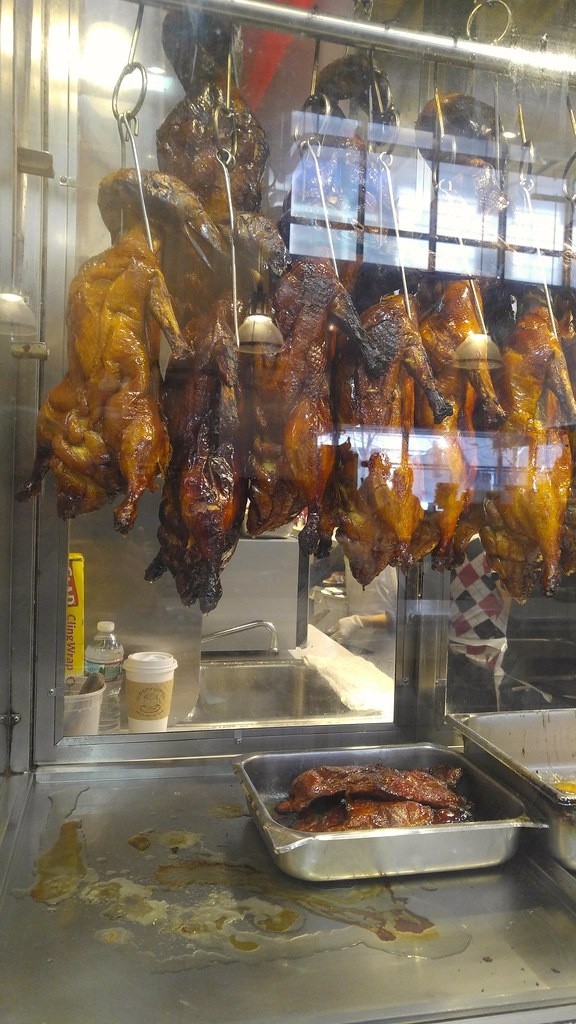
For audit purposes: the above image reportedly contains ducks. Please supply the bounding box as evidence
[10,11,576,614]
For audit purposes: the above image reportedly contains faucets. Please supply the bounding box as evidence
[200,619,278,654]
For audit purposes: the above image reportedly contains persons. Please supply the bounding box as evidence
[308,536,518,712]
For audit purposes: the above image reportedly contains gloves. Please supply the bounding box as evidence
[329,616,364,641]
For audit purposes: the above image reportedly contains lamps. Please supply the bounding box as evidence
[0,287,38,336]
[449,332,505,370]
[230,231,286,357]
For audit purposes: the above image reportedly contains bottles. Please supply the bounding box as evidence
[84,620,124,733]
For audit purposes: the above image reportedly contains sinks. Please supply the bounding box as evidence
[189,659,352,722]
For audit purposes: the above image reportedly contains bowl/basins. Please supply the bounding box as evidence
[65,677,106,736]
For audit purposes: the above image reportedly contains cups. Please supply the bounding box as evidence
[123,652,178,732]
